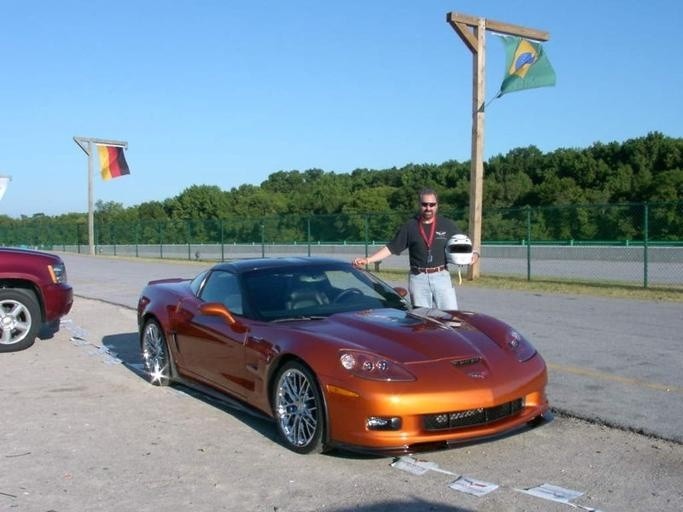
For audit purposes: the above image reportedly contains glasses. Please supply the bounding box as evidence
[420,202,437,207]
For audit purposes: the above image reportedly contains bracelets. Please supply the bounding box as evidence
[365,257,369,266]
[472,249,481,258]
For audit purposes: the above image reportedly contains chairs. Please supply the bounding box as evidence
[219,268,337,311]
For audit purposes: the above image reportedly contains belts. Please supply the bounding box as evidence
[410,266,446,275]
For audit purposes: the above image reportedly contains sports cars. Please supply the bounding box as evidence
[133,252,559,461]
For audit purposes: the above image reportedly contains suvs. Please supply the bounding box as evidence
[0,247,74,355]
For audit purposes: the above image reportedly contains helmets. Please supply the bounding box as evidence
[445,233,474,267]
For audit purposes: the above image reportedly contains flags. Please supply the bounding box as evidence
[95,144,130,181]
[496,35,557,98]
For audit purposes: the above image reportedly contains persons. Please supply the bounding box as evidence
[352,189,479,312]
